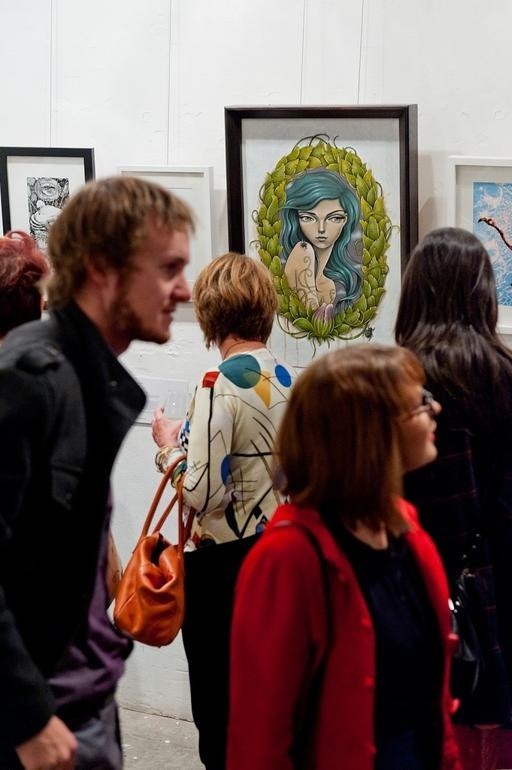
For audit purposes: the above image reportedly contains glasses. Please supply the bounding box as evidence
[401,389,433,421]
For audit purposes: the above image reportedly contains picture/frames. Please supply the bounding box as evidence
[444,153,512,336]
[0,146,213,306]
[224,103,421,370]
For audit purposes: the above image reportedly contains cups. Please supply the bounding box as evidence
[164,390,193,420]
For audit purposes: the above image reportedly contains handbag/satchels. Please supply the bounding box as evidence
[449,567,499,725]
[112,454,197,647]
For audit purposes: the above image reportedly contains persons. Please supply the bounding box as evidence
[389,226,511,770]
[1,172,202,769]
[1,229,52,339]
[150,252,301,768]
[223,341,463,770]
[280,167,364,318]
